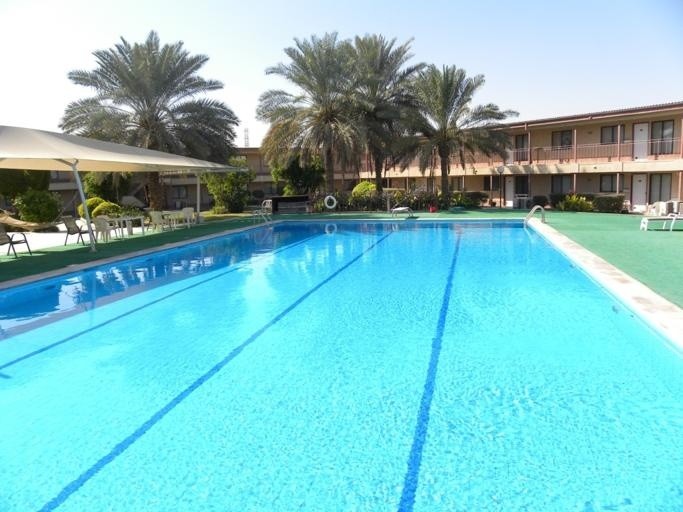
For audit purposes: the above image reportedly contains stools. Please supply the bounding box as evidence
[391,207,413,218]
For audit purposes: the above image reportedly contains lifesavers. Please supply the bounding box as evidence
[324,196,336,210]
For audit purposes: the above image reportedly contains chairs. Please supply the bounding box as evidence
[0,224,31,258]
[62,207,194,245]
[640,201,682,232]
